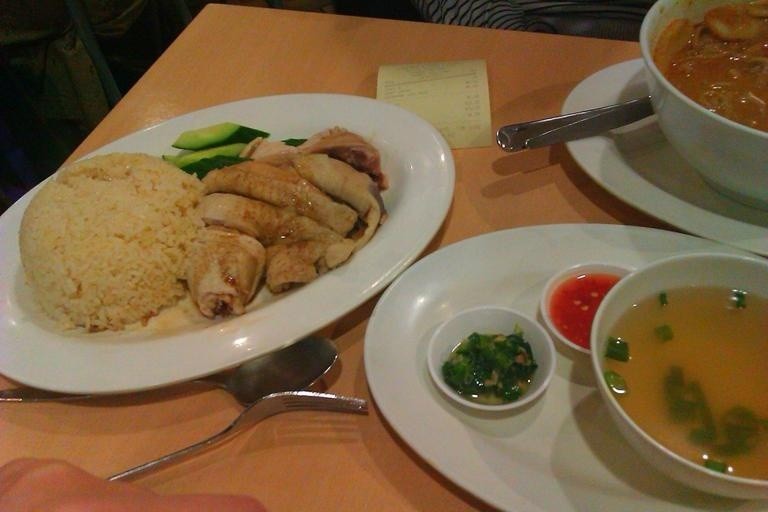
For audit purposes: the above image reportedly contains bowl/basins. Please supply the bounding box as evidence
[640,1,767,212]
[590,252,767,500]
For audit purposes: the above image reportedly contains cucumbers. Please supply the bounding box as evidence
[164,122,312,175]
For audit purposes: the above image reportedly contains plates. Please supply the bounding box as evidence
[363,223,768,512]
[0,92,457,396]
[561,57,768,261]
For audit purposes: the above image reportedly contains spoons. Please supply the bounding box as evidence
[0,336,338,406]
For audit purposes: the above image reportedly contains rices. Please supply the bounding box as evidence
[19,155,208,333]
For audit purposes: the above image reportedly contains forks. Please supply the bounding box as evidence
[104,392,369,484]
